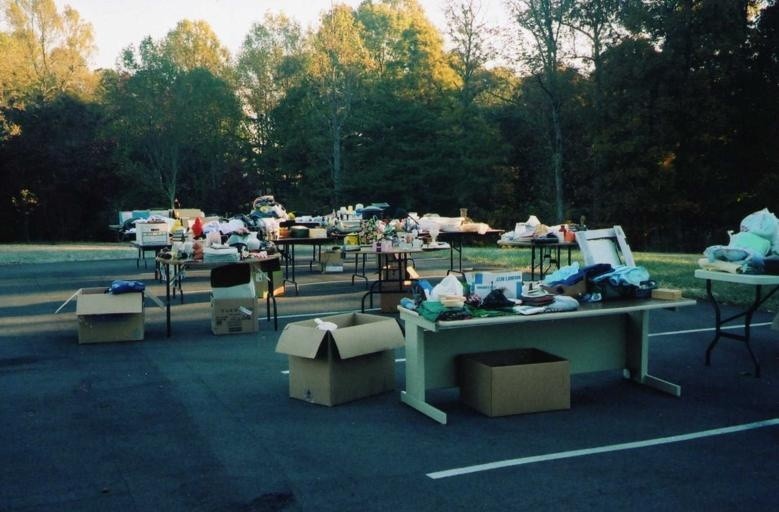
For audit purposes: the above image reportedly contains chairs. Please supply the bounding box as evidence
[569,226,639,270]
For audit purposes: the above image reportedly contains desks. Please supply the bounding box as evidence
[392,299,686,431]
[692,268,779,382]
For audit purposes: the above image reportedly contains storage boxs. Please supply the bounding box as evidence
[275,314,406,407]
[54,289,163,343]
[379,283,422,313]
[209,296,258,334]
[456,346,570,417]
